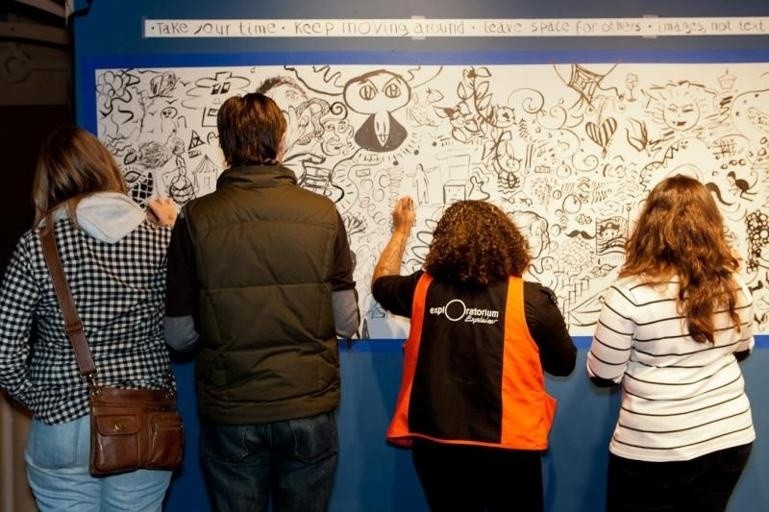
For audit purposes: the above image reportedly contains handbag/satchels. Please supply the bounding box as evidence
[89,386,184,477]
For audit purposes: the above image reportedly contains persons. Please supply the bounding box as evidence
[164,92,361,512]
[586,176,756,512]
[1,127,175,512]
[371,197,578,512]
